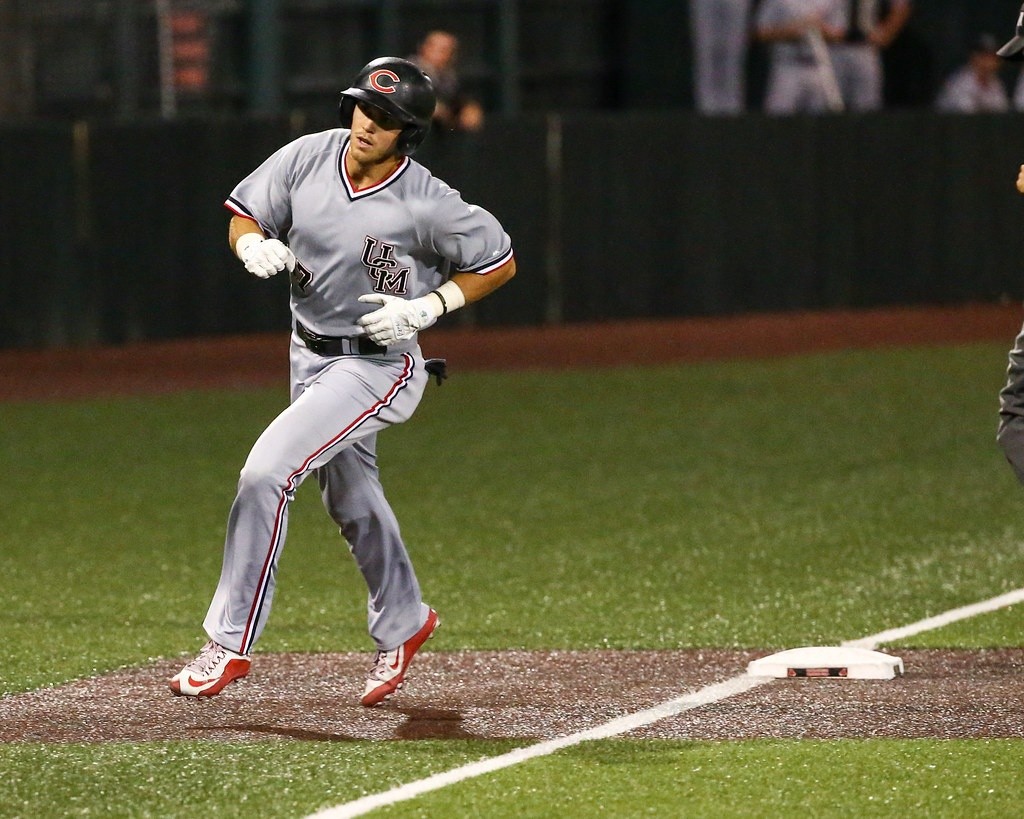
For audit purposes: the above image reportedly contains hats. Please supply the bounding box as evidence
[340,57,436,127]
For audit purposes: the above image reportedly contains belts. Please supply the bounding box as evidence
[297,320,387,356]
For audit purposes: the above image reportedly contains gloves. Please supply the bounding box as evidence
[357,293,438,346]
[236,233,296,279]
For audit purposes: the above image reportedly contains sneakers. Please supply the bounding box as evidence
[361,607,440,707]
[168,641,251,696]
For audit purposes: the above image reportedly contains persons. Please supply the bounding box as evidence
[167,56,517,706]
[996,164,1024,482]
[930,34,1008,112]
[414,28,484,127]
[753,0,907,113]
